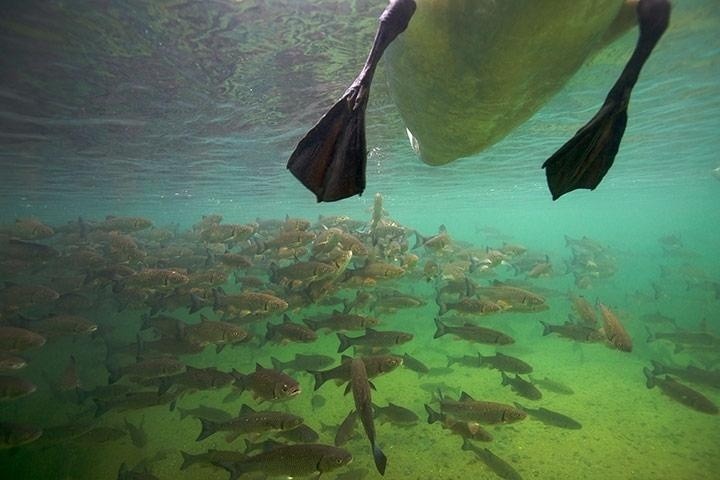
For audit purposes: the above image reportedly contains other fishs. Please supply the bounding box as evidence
[1,213,633,480]
